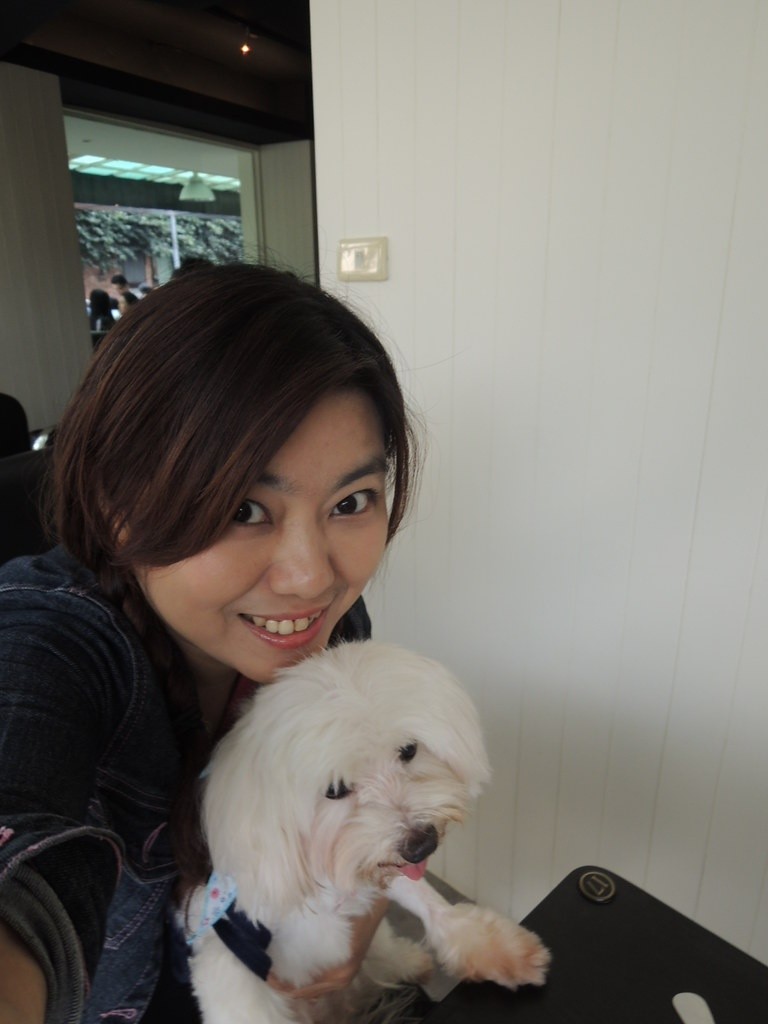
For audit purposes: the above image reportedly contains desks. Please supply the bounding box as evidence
[419,867,768,1024]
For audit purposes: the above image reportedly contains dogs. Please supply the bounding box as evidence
[163,639,550,1024]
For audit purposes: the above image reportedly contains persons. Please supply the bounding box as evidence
[110,274,129,309]
[0,259,426,1024]
[89,290,116,356]
[118,291,139,315]
